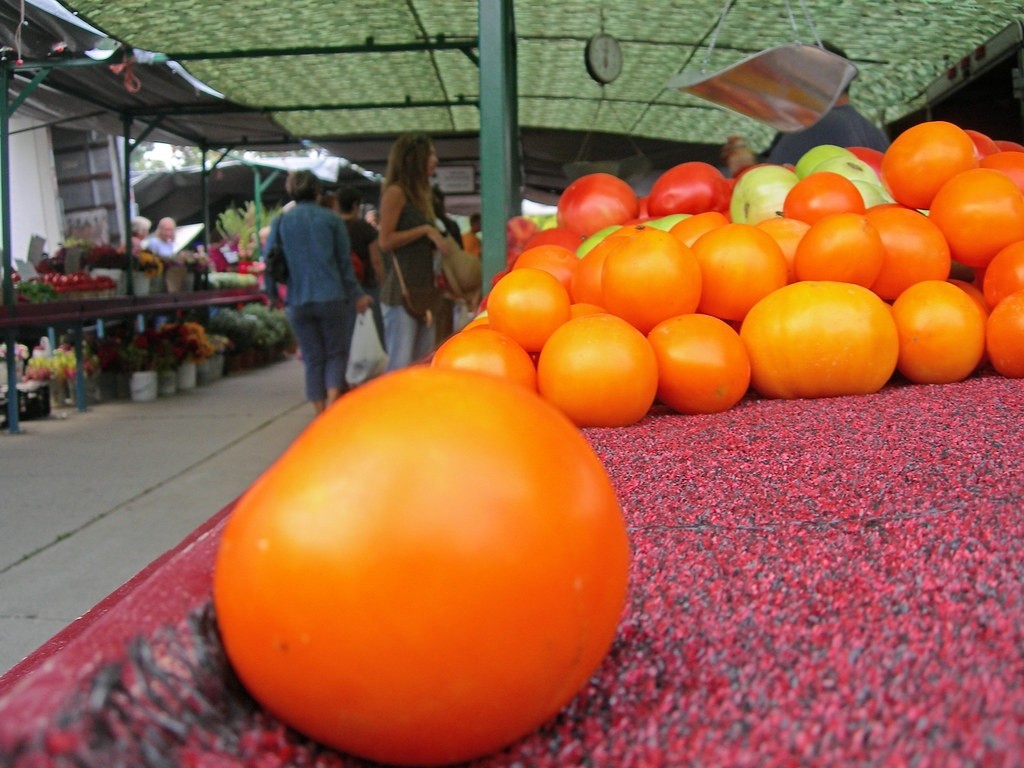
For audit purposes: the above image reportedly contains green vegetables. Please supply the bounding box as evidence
[13,280,64,304]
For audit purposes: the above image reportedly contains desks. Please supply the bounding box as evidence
[0,288,255,436]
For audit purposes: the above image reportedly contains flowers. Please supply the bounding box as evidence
[21,205,281,380]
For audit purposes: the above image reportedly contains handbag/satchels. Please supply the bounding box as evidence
[264,213,288,283]
[345,308,390,388]
[404,289,444,323]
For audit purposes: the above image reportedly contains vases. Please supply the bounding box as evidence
[160,370,176,393]
[130,369,156,400]
[93,267,149,297]
[197,356,223,384]
[166,270,186,292]
[176,362,196,389]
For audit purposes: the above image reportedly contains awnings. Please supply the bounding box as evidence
[0,0,307,434]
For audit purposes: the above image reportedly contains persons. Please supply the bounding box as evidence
[432,187,463,347]
[461,213,481,259]
[336,190,387,354]
[721,41,889,176]
[379,133,448,371]
[191,228,236,272]
[244,171,374,414]
[129,216,176,257]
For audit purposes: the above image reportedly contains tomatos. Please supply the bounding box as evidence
[211,365,628,766]
[11,271,118,292]
[425,119,1024,427]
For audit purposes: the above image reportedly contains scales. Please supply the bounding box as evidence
[668,0,858,135]
[562,0,653,186]
